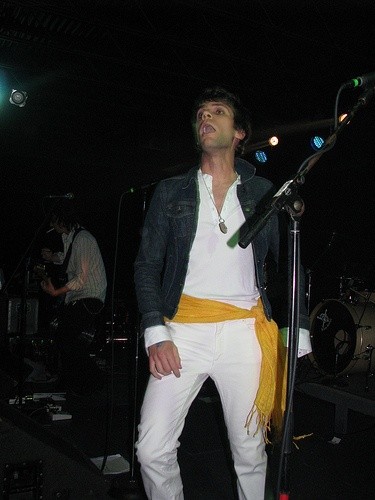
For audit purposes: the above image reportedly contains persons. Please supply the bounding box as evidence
[40,206,109,393]
[133,87,312,500]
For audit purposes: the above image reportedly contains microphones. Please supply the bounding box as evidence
[126,179,159,194]
[351,71,375,87]
[48,192,76,200]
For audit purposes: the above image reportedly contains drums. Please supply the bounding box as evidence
[354,289,375,303]
[307,299,375,377]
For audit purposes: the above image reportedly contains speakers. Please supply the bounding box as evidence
[0,400,111,500]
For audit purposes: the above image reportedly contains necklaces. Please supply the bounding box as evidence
[203,171,239,233]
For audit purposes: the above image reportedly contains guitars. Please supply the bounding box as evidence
[33,263,68,307]
